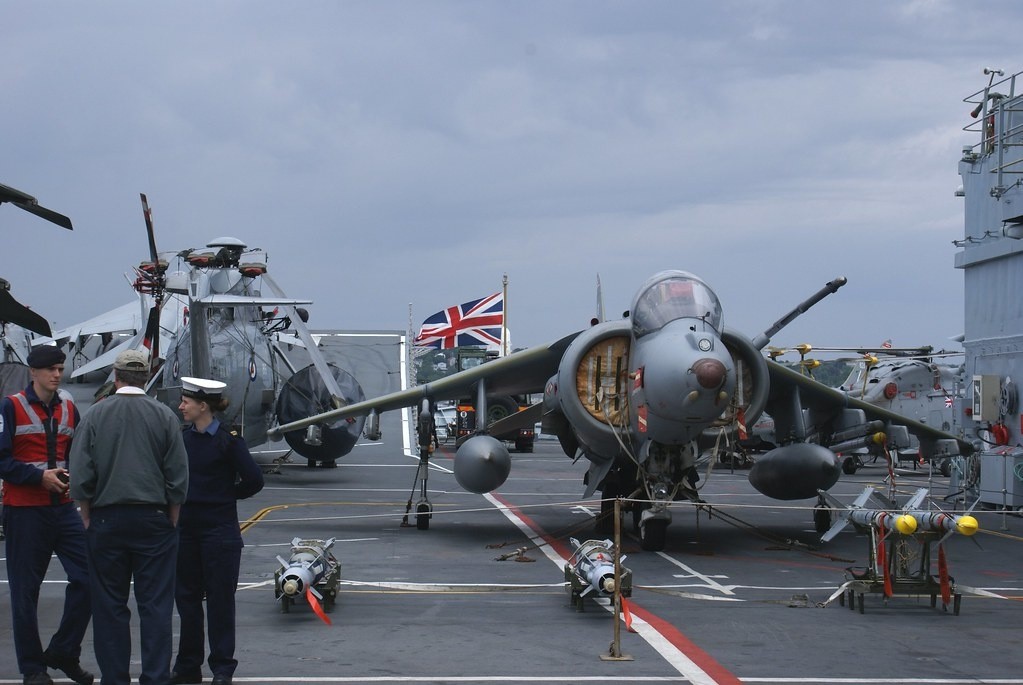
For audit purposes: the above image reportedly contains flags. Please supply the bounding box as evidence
[413,291,504,347]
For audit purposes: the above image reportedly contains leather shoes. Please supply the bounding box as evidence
[212,674,232,685]
[170,669,202,684]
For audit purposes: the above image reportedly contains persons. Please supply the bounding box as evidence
[644,277,714,329]
[70,349,189,685]
[0,345,94,685]
[170,376,267,685]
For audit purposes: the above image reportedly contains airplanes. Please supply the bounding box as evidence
[25,193,369,469]
[267,270,977,551]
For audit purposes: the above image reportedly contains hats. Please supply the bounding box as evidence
[27,345,67,369]
[113,348,150,371]
[179,376,227,401]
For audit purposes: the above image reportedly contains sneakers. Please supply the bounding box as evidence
[44,646,94,683]
[23,670,54,685]
